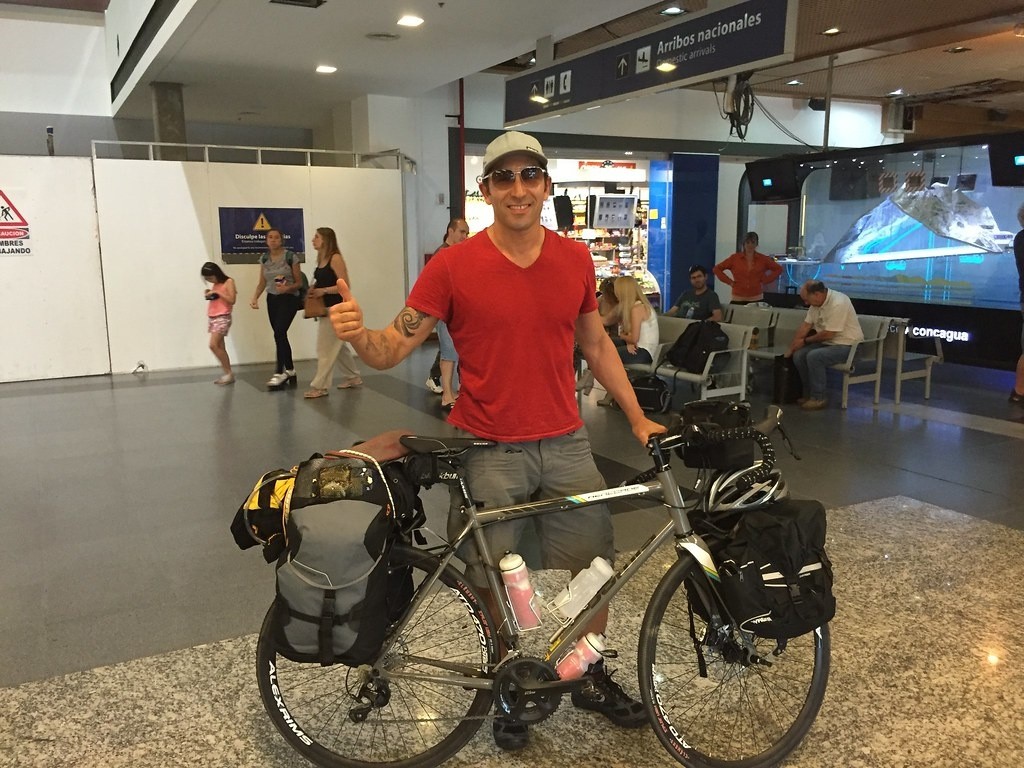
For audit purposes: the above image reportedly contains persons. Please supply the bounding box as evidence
[426,219,461,407]
[328,131,671,749]
[784,280,863,410]
[250,229,304,388]
[573,276,660,406]
[304,227,362,398]
[665,265,722,323]
[713,232,783,304]
[202,262,236,385]
[1010,206,1024,400]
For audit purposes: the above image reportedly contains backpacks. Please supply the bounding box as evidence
[262,249,310,310]
[665,320,731,373]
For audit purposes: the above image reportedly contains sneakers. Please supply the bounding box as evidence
[573,667,649,728]
[425,376,443,394]
[494,692,528,751]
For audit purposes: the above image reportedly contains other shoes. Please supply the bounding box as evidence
[337,379,363,389]
[802,398,827,409]
[795,396,809,403]
[1009,388,1024,405]
[214,375,236,385]
[304,389,329,398]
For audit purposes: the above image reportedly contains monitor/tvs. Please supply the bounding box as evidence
[746,157,801,201]
[592,196,637,228]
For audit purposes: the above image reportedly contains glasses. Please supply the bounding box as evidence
[483,167,547,190]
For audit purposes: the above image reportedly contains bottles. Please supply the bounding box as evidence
[500,550,540,628]
[554,555,614,618]
[555,632,605,680]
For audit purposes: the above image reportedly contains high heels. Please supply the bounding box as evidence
[596,392,614,405]
[267,372,289,387]
[286,370,297,385]
[575,370,595,396]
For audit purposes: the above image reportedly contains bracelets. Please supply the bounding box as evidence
[324,288,328,295]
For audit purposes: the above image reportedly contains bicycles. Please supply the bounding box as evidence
[256,403,833,768]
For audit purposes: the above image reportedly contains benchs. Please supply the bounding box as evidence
[720,303,894,410]
[882,316,941,406]
[577,315,753,401]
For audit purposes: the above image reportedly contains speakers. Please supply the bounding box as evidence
[807,98,826,111]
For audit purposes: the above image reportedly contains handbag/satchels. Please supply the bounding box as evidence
[305,288,328,318]
[687,498,837,640]
[775,355,802,404]
[632,376,671,412]
[233,438,423,667]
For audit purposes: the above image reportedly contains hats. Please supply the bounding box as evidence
[483,131,549,179]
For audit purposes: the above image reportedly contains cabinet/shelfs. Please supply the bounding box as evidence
[558,203,615,239]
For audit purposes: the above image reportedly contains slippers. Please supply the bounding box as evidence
[441,401,455,411]
